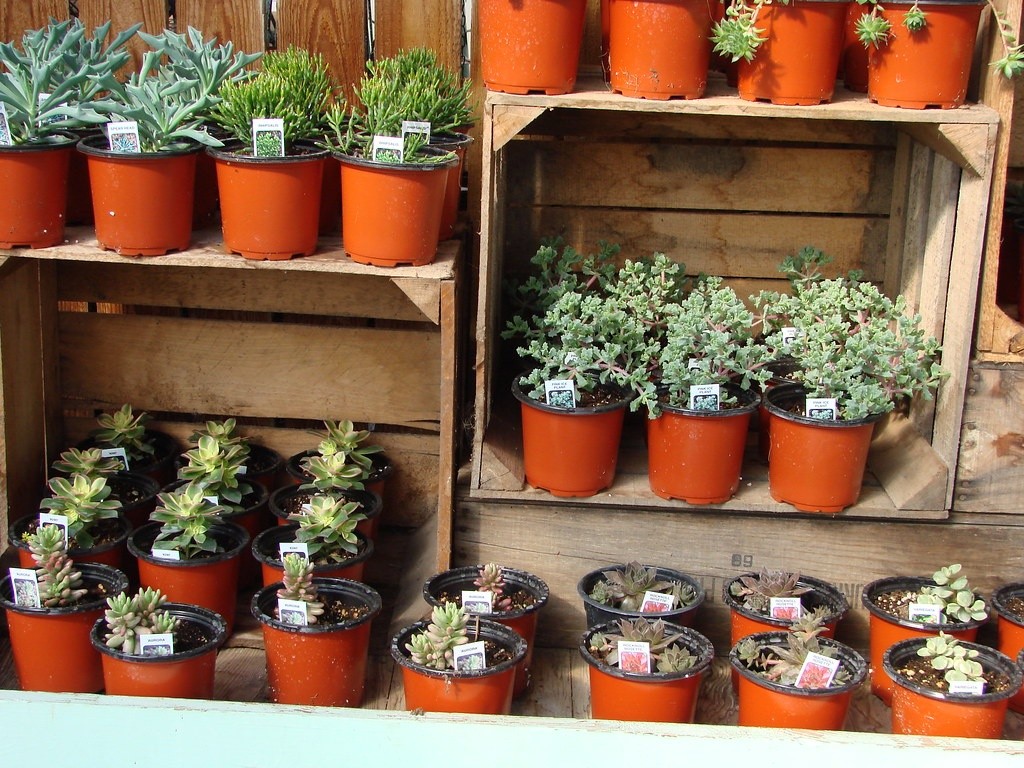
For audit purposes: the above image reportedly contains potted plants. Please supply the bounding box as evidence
[0,0,473,267]
[506,241,944,512]
[390,618,527,716]
[730,630,866,735]
[1,406,386,709]
[581,619,712,725]
[990,582,1024,659]
[718,0,849,106]
[884,638,1023,742]
[863,0,990,112]
[860,575,984,704]
[725,572,849,648]
[421,566,548,697]
[581,563,707,628]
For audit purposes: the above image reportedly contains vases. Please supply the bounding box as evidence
[601,0,714,100]
[477,0,587,96]
